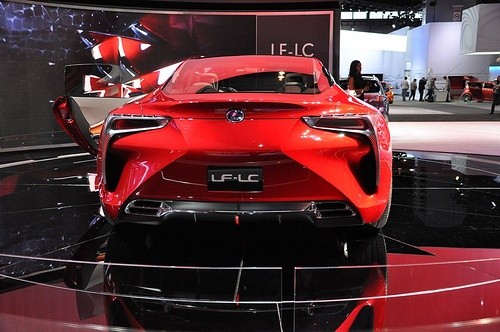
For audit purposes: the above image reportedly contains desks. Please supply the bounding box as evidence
[425,90,448,101]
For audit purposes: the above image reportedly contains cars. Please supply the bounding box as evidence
[381,82,394,104]
[460,80,497,102]
[63,220,388,332]
[340,74,389,125]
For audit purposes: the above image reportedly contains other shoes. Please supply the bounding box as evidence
[409,97,410,100]
[489,112,493,114]
[412,99,415,101]
[419,99,424,101]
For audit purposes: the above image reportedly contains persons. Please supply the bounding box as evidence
[418,78,428,102]
[443,75,451,102]
[424,78,439,102]
[488,75,500,114]
[348,60,369,102]
[400,77,409,101]
[409,79,417,101]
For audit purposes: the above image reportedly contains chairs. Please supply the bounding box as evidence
[285,73,307,87]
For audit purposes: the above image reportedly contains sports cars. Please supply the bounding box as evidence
[52,54,394,229]
